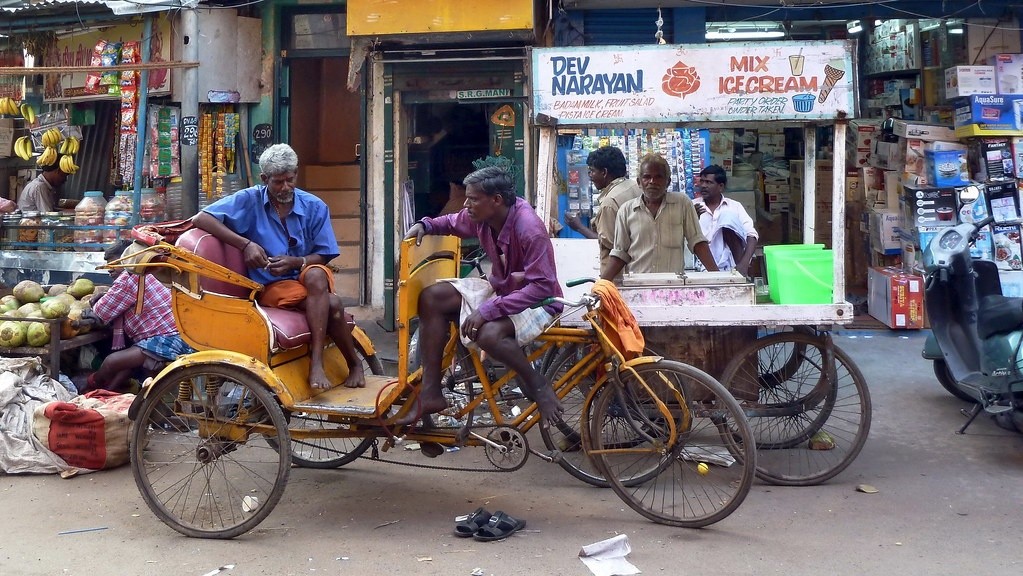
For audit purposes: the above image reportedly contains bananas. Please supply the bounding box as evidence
[0,96,20,116]
[59,155,79,173]
[36,146,58,167]
[41,128,61,148]
[20,103,35,124]
[14,136,32,161]
[60,135,80,154]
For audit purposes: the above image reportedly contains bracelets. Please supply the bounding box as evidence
[242,241,252,251]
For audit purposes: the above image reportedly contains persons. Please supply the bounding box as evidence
[600,153,720,282]
[72,235,193,389]
[691,164,759,276]
[191,142,366,390]
[564,146,643,279]
[393,163,564,429]
[18,153,68,212]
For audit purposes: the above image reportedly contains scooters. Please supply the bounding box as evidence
[914,183,1023,437]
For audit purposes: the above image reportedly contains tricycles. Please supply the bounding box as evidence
[90,217,757,540]
[420,244,874,488]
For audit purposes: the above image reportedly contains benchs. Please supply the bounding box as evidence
[172,228,356,367]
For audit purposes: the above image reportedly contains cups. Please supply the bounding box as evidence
[242,494,259,513]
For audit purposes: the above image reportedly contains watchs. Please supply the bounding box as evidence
[300,256,307,272]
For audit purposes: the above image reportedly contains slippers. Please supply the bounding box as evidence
[454,507,493,536]
[473,511,525,541]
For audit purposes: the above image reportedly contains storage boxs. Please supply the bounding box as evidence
[0,117,30,157]
[709,19,1022,329]
[18,170,43,202]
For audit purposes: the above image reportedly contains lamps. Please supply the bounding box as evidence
[705,21,785,40]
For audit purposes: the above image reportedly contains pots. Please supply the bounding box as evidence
[939,164,958,177]
[936,206,953,221]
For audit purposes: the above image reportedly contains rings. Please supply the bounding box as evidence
[472,327,477,331]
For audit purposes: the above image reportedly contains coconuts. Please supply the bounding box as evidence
[0,278,103,347]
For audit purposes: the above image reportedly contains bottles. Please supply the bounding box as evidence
[1,212,73,249]
[140,189,165,226]
[199,174,245,211]
[167,177,182,221]
[73,191,133,251]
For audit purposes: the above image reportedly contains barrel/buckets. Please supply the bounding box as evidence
[762,244,832,308]
